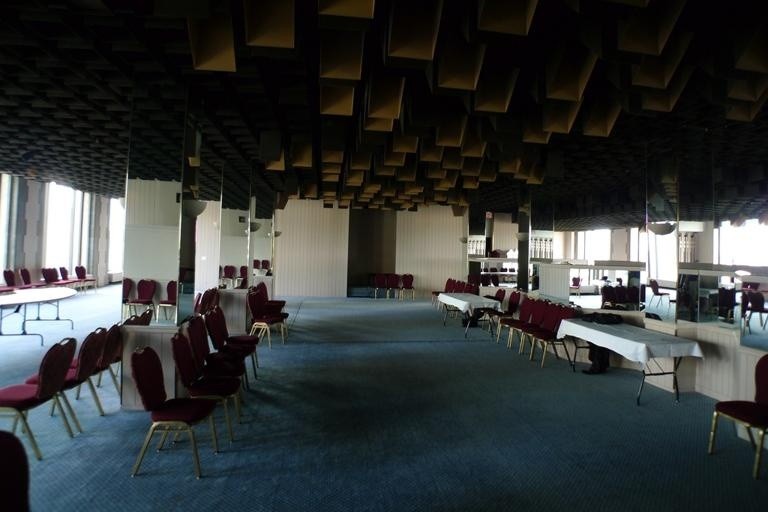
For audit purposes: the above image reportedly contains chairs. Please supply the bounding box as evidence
[0,336,76,459]
[159,301,260,448]
[430,260,768,406]
[29,307,156,416]
[1,259,98,303]
[190,257,295,346]
[128,344,221,482]
[707,353,767,480]
[368,271,417,302]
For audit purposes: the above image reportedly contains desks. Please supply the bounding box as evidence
[0,285,78,345]
